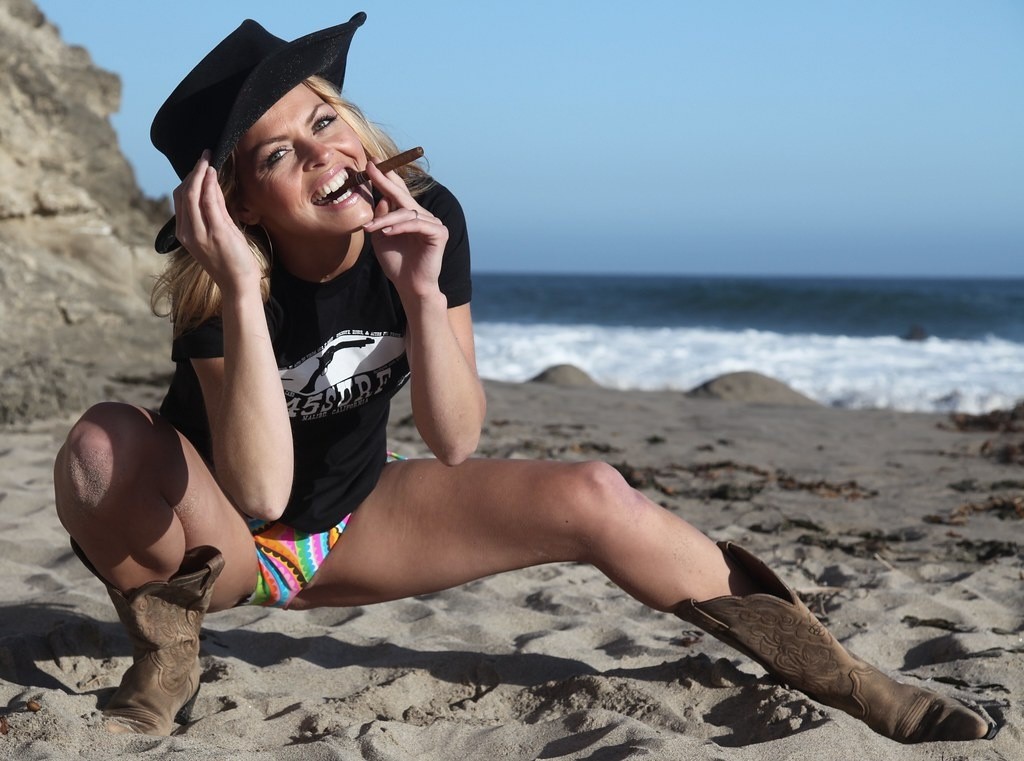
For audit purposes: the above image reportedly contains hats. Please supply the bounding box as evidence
[150,12,367,255]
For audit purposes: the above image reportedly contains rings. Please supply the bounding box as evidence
[411,209,419,219]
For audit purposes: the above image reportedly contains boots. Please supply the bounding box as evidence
[69,534,226,738]
[672,539,998,744]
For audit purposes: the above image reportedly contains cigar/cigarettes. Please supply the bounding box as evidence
[344,147,425,191]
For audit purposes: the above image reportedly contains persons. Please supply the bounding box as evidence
[54,11,990,745]
